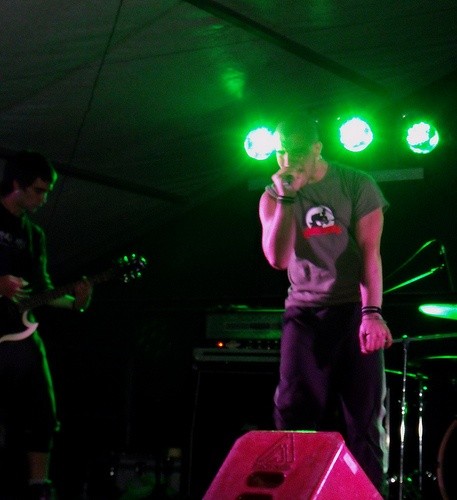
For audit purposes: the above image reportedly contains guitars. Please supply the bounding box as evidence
[0,253,147,344]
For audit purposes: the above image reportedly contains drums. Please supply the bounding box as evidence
[436,418,457,500]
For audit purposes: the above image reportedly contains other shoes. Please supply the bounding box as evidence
[26,481,52,500]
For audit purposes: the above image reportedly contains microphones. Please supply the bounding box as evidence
[283,175,293,184]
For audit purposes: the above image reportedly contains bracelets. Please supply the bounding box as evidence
[277,195,297,203]
[73,300,76,310]
[361,306,382,314]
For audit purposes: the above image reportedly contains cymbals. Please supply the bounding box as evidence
[417,303,457,320]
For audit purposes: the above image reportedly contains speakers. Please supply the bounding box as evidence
[203,430,383,500]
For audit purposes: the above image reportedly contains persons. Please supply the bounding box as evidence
[0,152,92,500]
[259,112,393,500]
